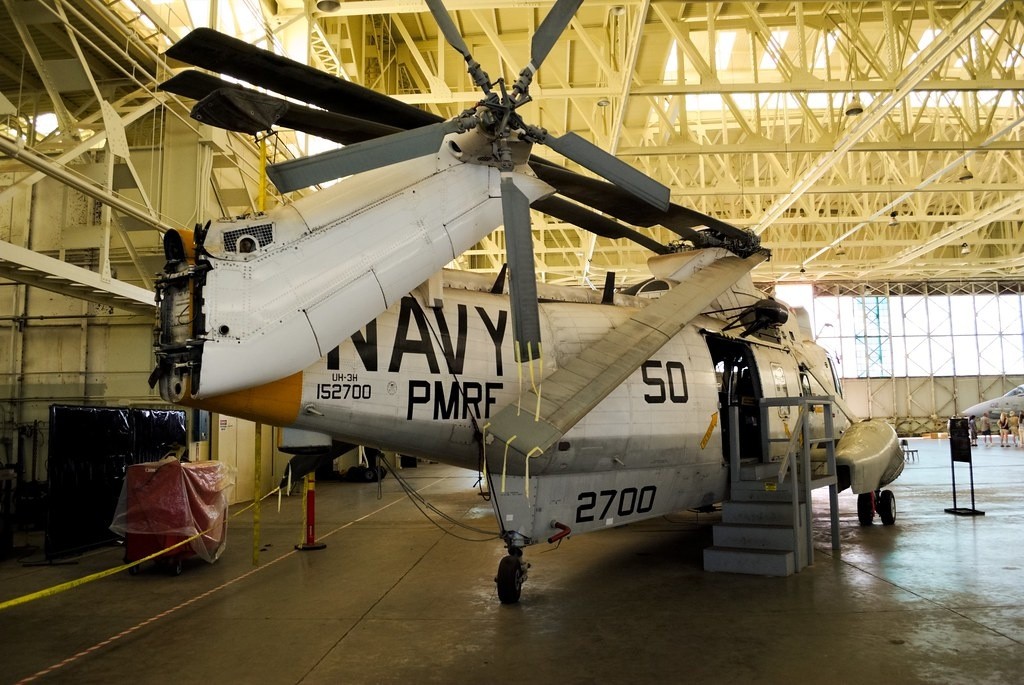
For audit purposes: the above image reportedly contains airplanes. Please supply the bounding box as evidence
[147,0,920,612]
[959,384,1024,422]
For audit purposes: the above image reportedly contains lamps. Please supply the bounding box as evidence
[316,0,341,12]
[886,157,900,226]
[957,112,974,181]
[800,260,808,281]
[596,97,610,107]
[845,23,863,116]
[836,203,846,256]
[961,236,971,254]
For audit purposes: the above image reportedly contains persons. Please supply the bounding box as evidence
[1018,414,1024,447]
[969,415,979,446]
[980,412,995,447]
[1008,411,1020,447]
[997,412,1011,447]
